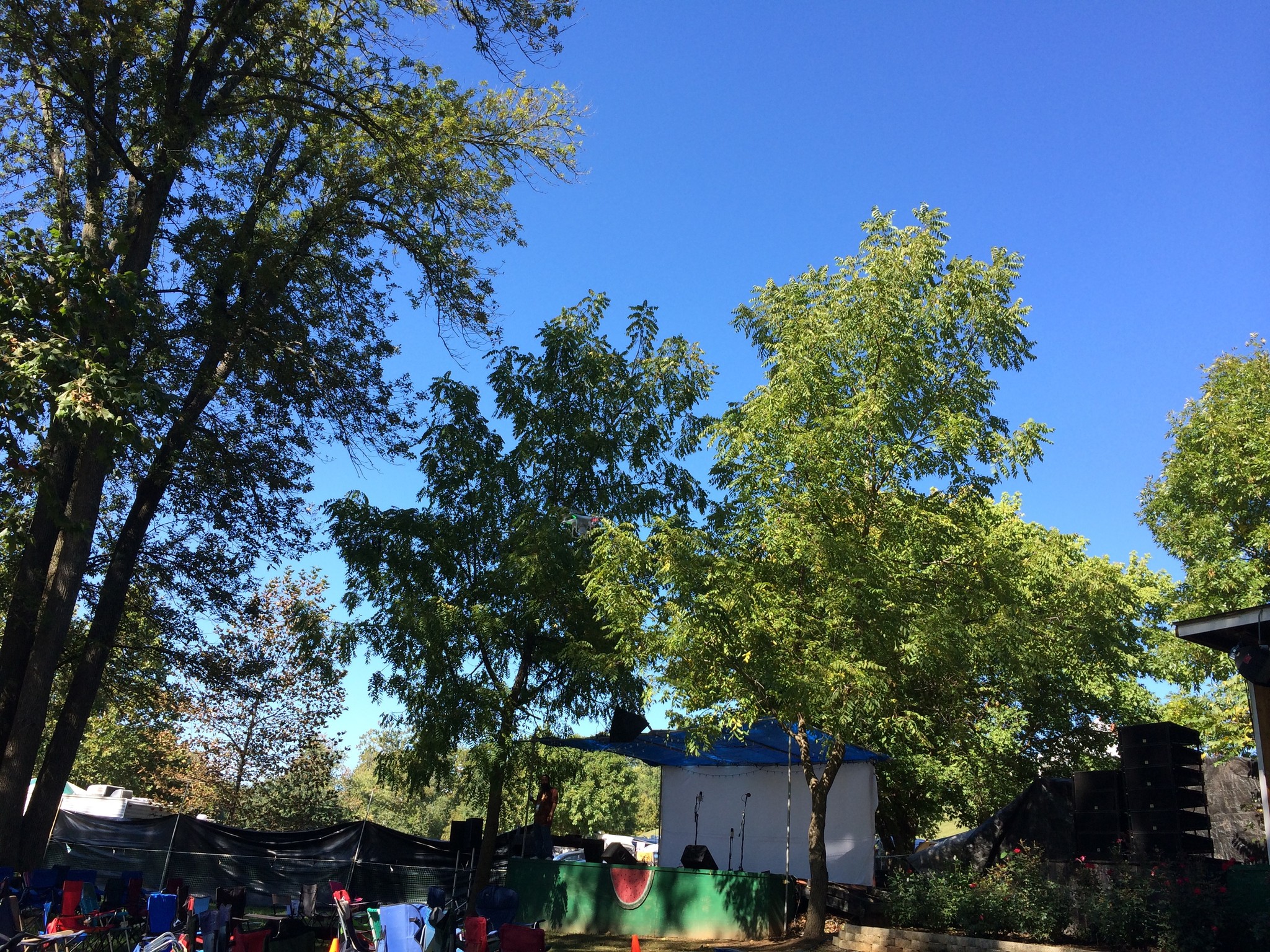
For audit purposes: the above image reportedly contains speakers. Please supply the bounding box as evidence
[680,844,718,871]
[466,818,484,843]
[449,819,468,849]
[601,842,638,865]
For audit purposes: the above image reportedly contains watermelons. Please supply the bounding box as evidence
[610,868,655,909]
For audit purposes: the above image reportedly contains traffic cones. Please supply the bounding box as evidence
[630,934,642,952]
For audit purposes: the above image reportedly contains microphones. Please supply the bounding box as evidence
[699,791,702,801]
[745,793,751,797]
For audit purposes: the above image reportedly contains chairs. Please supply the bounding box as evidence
[0,869,295,952]
[300,878,548,952]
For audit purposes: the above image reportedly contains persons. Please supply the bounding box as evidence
[528,775,557,860]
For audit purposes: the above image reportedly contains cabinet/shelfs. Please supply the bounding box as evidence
[1074,721,1214,857]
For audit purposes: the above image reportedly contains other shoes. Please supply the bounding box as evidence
[545,857,552,860]
[530,855,539,860]
